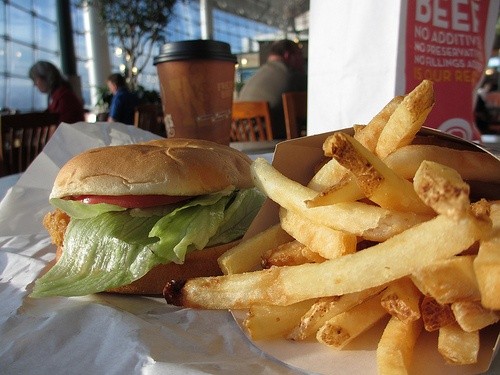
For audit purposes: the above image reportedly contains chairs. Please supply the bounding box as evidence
[134,107,156,131]
[0,114,60,178]
[282,92,307,140]
[230,101,274,142]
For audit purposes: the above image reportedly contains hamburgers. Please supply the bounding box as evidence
[28,137,266,299]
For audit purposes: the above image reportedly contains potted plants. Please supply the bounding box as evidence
[94,85,112,122]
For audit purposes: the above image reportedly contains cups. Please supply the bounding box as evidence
[154,39,238,145]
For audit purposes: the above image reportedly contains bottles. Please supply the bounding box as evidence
[472,1,500,148]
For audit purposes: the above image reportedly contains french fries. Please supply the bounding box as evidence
[164,77,500,375]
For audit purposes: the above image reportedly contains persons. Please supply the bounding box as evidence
[237,38,306,139]
[108,73,139,129]
[29,60,85,170]
